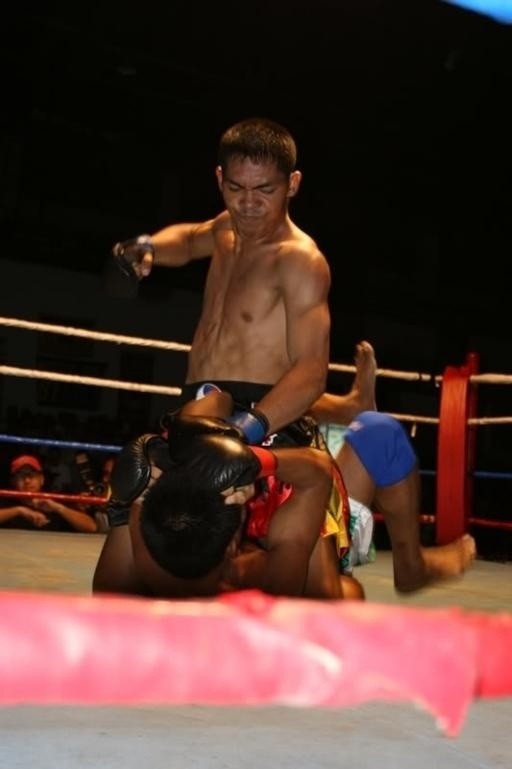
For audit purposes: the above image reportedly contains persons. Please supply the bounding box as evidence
[110,117,373,606]
[90,337,481,605]
[2,443,129,534]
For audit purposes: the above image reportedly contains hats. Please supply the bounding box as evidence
[11,455,42,474]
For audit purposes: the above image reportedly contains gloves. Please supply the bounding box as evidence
[107,405,277,527]
